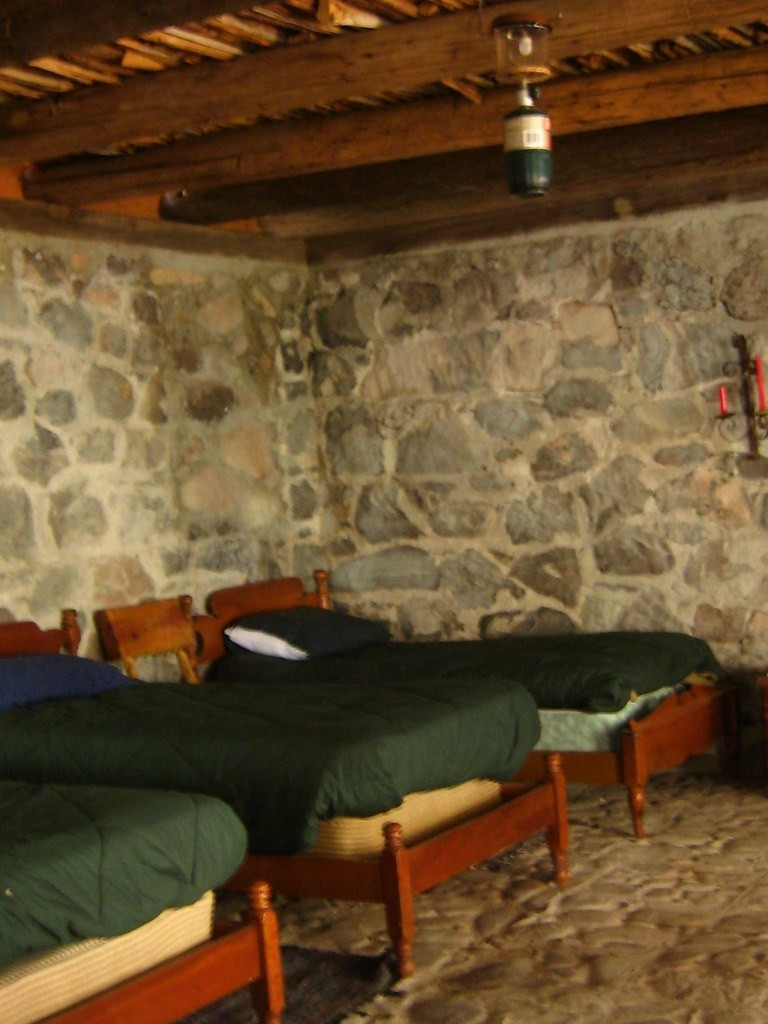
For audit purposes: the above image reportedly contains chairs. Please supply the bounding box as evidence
[94,598,201,684]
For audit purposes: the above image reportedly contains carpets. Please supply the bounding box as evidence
[185,940,407,1024]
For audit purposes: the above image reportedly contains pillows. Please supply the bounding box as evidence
[0,653,131,711]
[221,603,391,661]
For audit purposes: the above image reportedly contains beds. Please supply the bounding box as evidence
[0,778,289,1024]
[207,569,734,838]
[0,608,570,978]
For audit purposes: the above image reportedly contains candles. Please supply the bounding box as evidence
[720,384,727,414]
[755,355,768,411]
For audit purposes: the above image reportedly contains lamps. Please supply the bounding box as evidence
[475,0,557,196]
